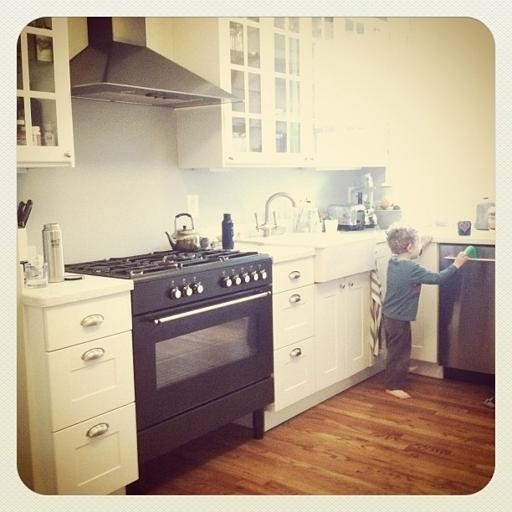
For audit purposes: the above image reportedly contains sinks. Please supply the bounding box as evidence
[234,231,378,284]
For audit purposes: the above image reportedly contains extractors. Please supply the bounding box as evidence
[69,17,244,110]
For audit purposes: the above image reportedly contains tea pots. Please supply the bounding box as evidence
[166,212,197,251]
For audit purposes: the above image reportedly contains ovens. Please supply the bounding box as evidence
[132,285,275,466]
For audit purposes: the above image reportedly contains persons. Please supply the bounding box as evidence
[376,220,471,400]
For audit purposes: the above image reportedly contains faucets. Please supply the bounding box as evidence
[263,192,296,236]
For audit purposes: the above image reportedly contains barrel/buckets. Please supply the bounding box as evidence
[373,210,403,230]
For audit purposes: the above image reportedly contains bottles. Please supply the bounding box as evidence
[221,214,234,249]
[487,202,496,232]
[200,238,209,251]
[42,223,65,284]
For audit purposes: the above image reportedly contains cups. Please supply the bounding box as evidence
[25,262,48,289]
[325,219,338,234]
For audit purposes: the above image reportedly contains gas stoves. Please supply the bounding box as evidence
[63,248,273,316]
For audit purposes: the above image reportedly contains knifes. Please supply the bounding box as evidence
[17,199,33,228]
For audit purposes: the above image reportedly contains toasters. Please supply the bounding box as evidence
[328,203,367,231]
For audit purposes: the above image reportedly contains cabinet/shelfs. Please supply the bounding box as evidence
[220,15,307,164]
[43,292,140,496]
[273,258,312,403]
[16,16,74,166]
[316,272,370,389]
